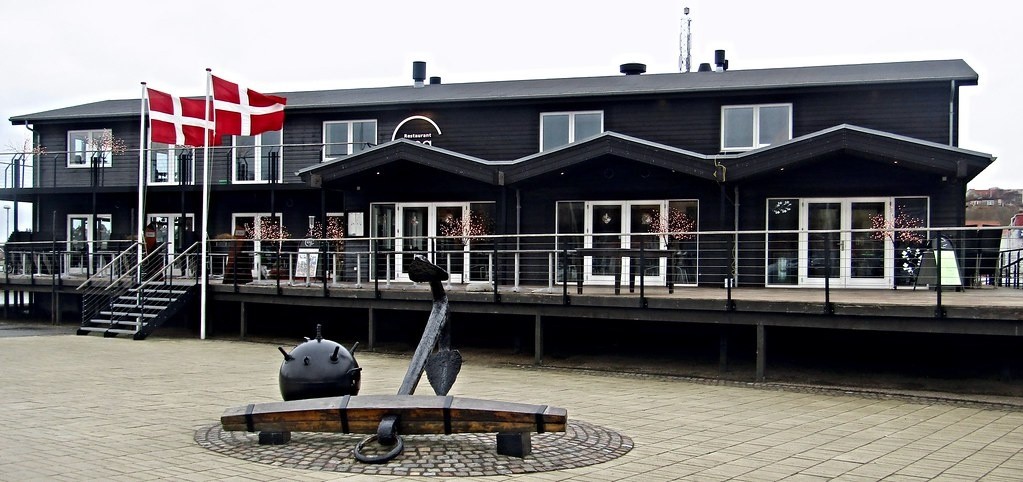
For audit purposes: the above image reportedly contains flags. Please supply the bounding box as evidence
[210,74,287,146]
[146,86,223,149]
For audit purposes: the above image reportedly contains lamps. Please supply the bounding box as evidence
[714,49,726,73]
[412,61,427,89]
